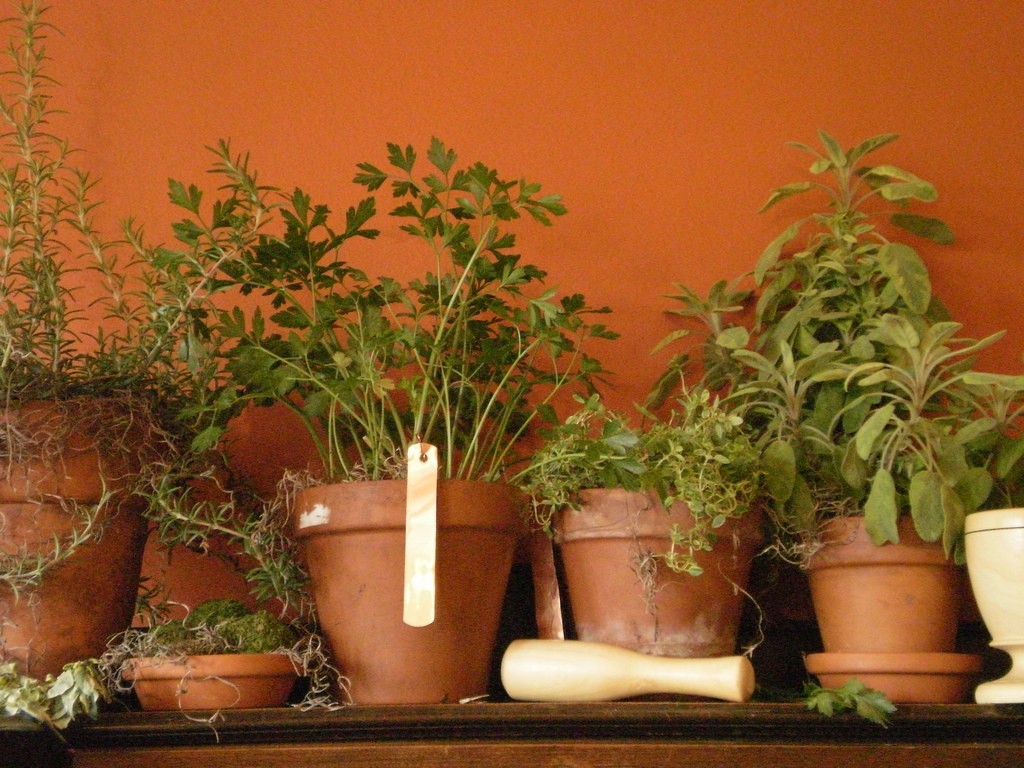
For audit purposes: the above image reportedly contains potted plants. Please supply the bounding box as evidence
[961,429,1024,705]
[500,636,901,729]
[0,0,350,737]
[119,598,303,733]
[149,132,619,704]
[525,393,773,700]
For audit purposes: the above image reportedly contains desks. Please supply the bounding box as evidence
[1,701,1023,766]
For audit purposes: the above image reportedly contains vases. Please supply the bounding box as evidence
[641,132,1024,703]
